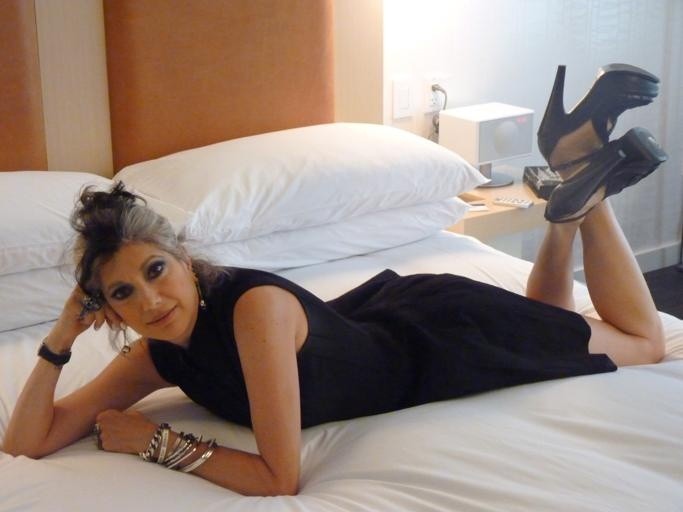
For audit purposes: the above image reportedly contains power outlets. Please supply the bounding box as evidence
[424,81,443,115]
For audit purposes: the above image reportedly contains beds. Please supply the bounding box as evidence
[1,226,683,510]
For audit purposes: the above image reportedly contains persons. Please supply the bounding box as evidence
[1,58,671,496]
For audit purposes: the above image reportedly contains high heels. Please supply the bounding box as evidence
[544,127,669,223]
[537,63,659,172]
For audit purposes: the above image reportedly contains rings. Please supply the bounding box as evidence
[79,308,90,319]
[83,295,102,310]
[95,421,99,436]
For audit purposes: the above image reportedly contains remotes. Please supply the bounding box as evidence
[493,196,533,209]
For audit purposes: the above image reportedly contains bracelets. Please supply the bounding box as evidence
[37,344,74,366]
[140,417,219,478]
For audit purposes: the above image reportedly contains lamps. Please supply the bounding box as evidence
[437,101,534,190]
